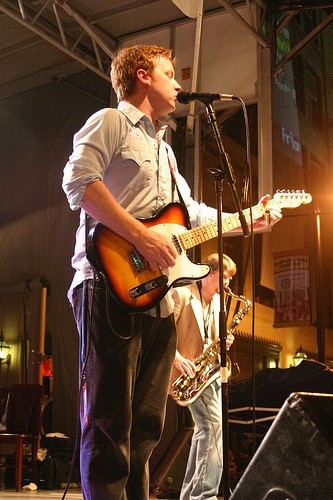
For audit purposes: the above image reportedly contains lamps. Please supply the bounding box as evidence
[0,331,11,367]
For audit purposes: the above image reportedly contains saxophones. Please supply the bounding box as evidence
[171,286,252,406]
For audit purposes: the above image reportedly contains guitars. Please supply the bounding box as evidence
[86,188,313,311]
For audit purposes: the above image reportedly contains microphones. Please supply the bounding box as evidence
[177,91,240,104]
[208,168,226,179]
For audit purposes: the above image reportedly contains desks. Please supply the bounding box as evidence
[0,433,33,492]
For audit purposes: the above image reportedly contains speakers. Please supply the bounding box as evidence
[229,391,333,500]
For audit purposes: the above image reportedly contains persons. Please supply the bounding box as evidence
[62,45,284,500]
[168,251,238,500]
